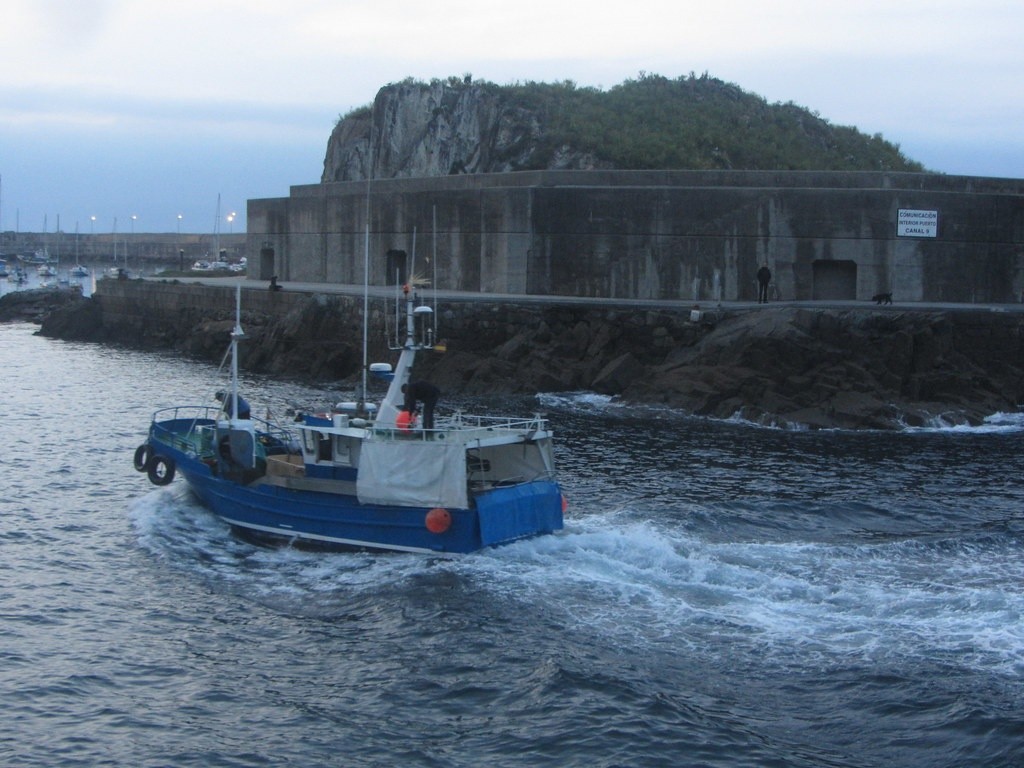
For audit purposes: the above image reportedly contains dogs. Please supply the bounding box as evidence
[872,292,893,306]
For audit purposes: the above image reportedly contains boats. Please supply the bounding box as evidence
[40,278,85,295]
[17,248,59,263]
[0,259,14,275]
[37,264,59,276]
[69,263,91,276]
[7,266,29,282]
[191,256,247,272]
[105,266,129,279]
[134,134,567,560]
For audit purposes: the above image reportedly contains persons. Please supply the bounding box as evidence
[401,382,439,436]
[215,391,251,420]
[755,261,772,303]
